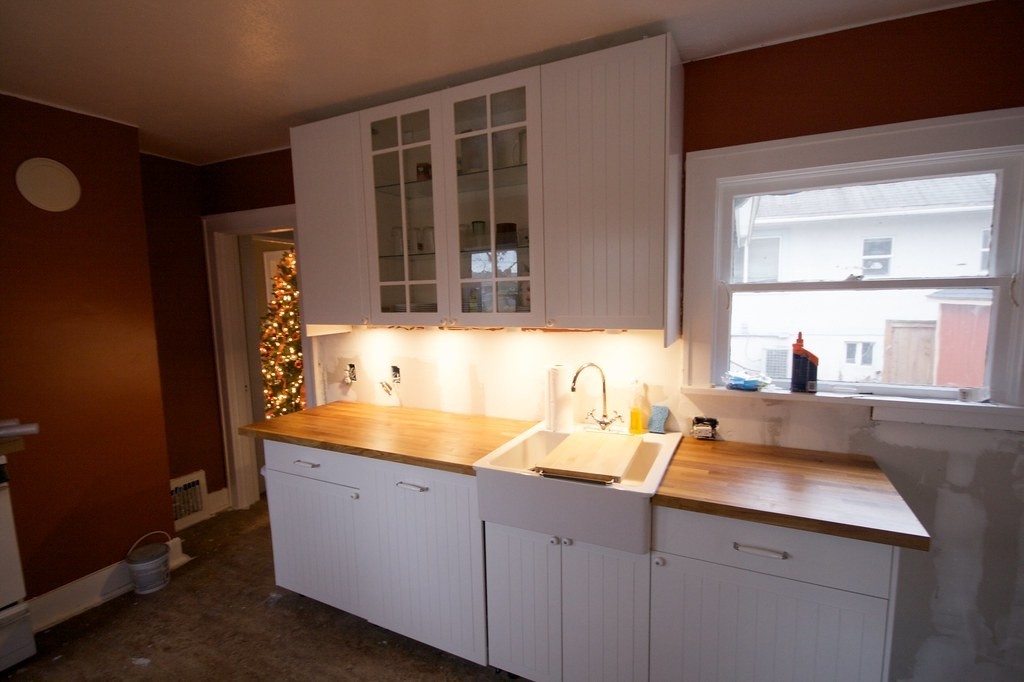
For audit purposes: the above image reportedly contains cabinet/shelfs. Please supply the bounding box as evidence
[0,421,40,672]
[237,402,933,682]
[289,32,685,348]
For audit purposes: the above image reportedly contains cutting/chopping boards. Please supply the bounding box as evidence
[536,430,644,483]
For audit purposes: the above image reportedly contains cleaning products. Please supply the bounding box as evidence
[789,331,818,392]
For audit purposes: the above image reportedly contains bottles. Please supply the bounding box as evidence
[416,148,430,181]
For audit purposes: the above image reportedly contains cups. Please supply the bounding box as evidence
[460,225,472,248]
[395,227,434,254]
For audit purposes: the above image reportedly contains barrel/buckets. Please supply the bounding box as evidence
[127,529,171,596]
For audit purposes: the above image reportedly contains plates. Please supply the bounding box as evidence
[391,303,437,311]
[461,297,478,312]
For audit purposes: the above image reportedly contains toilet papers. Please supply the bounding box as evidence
[545,362,574,431]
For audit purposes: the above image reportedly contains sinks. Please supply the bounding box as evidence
[471,420,685,555]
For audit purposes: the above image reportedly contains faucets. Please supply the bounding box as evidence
[571,364,607,418]
